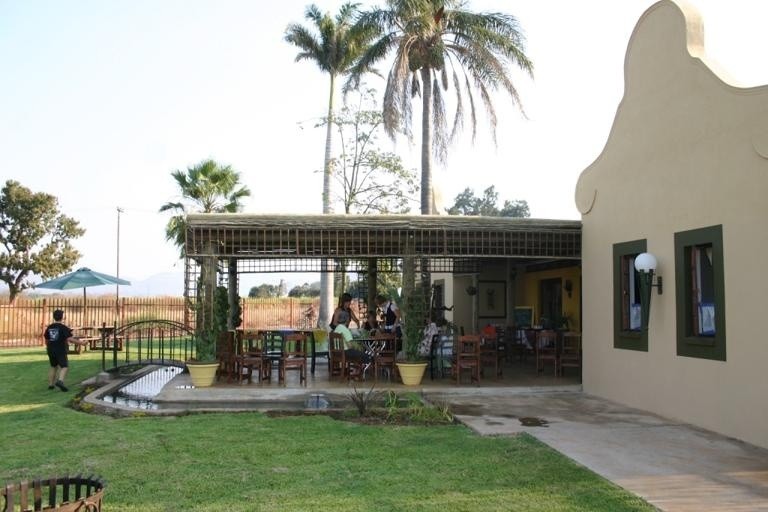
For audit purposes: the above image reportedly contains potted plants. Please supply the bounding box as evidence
[395,282,429,386]
[186,327,219,388]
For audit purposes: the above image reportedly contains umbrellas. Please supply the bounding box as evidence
[32,265,131,327]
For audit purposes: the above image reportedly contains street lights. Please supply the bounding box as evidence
[116,205,125,298]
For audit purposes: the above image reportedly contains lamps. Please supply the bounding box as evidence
[634,252,664,299]
[565,278,575,301]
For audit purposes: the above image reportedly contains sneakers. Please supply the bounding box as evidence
[55,380,68,392]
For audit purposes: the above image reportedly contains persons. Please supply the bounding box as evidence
[43,309,88,393]
[328,292,360,372]
[301,302,317,328]
[362,310,379,329]
[417,316,439,357]
[374,294,404,356]
[332,311,373,364]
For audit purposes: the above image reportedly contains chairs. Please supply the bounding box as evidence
[68,323,126,354]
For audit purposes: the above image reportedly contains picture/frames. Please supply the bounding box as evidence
[473,279,510,319]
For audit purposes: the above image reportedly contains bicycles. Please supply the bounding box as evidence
[295,312,318,330]
